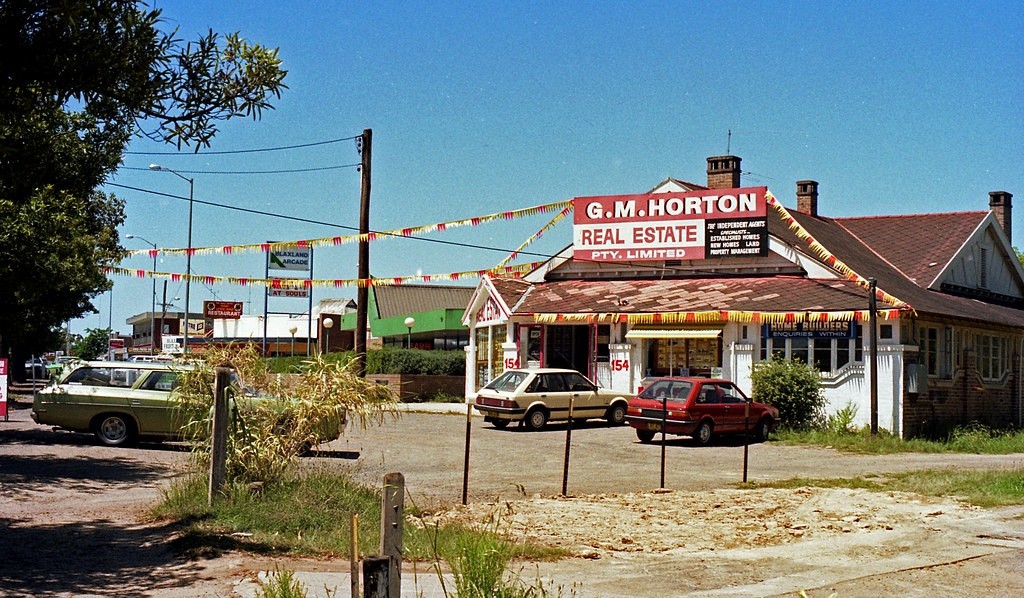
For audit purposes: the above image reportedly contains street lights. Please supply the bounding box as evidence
[126,236,157,355]
[404,317,415,348]
[160,296,180,349]
[322,318,334,355]
[289,325,298,357]
[148,164,193,354]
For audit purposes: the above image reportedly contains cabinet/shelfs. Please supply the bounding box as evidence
[657,339,718,368]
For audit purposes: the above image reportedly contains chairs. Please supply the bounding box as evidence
[677,387,690,398]
[529,377,549,392]
[705,389,719,403]
[549,377,563,392]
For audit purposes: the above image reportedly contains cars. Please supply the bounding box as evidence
[31,360,346,455]
[93,355,178,379]
[623,375,778,447]
[474,368,637,432]
[46,356,83,379]
[26,355,50,378]
